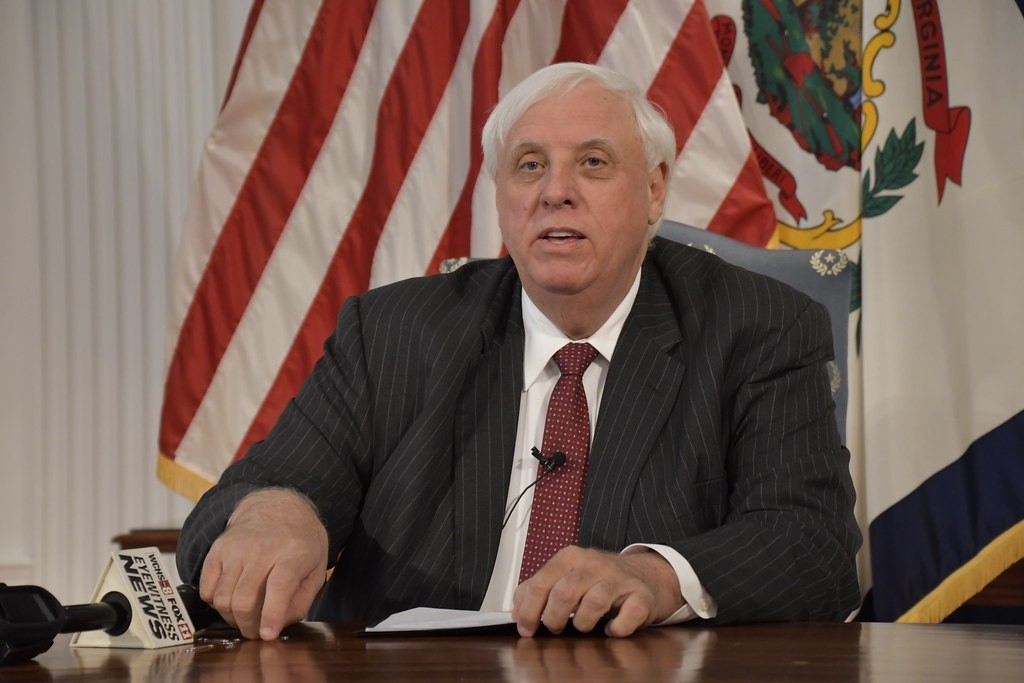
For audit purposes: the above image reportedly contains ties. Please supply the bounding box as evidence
[518,343,598,585]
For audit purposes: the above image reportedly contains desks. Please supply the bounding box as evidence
[0,621,1024,683]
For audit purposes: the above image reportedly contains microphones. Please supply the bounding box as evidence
[0,547,215,665]
[544,452,567,474]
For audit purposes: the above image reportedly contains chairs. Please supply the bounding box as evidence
[440,221,852,457]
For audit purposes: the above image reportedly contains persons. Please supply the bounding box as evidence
[196,638,863,683]
[174,60,864,637]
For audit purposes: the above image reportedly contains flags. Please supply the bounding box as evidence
[152,0,779,501]
